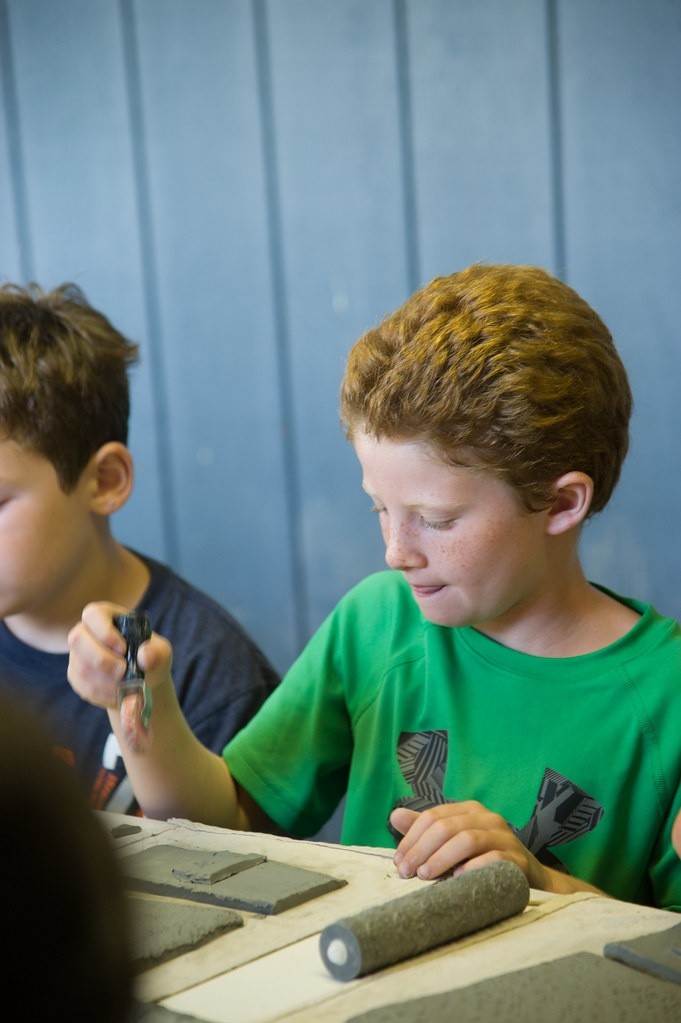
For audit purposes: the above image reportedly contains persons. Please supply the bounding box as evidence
[0,279,281,816]
[65,264,681,914]
